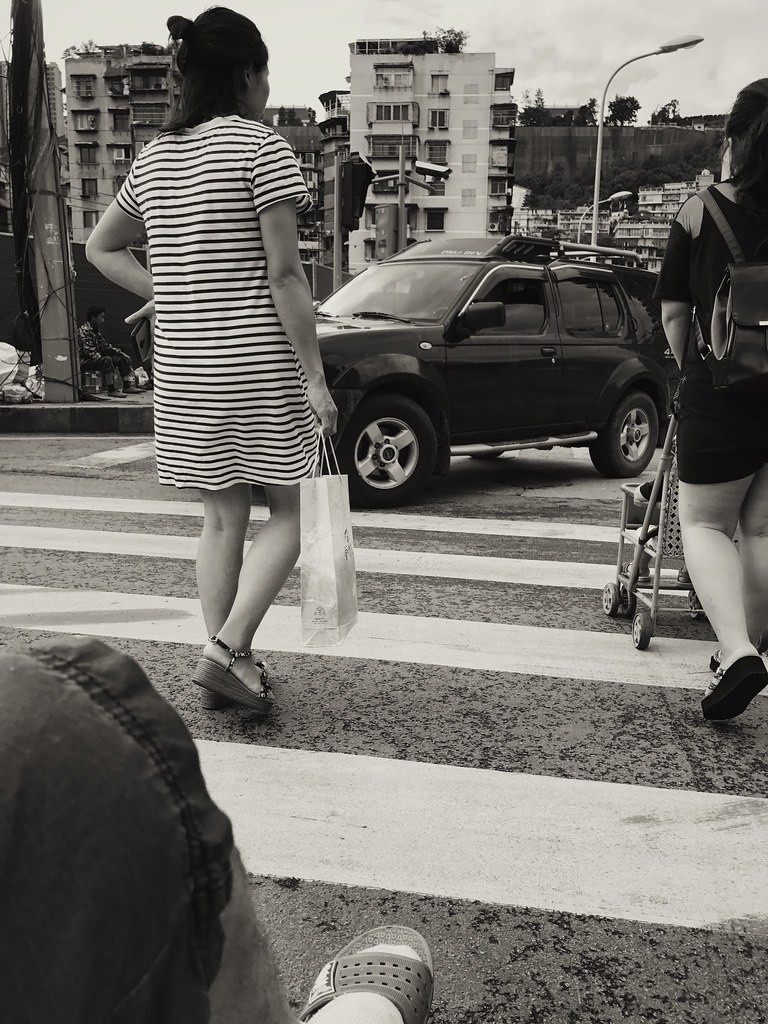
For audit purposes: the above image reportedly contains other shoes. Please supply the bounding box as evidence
[108,389,128,398]
[122,386,147,393]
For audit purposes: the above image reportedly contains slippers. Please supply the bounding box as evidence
[701,648,768,720]
[298,926,434,1024]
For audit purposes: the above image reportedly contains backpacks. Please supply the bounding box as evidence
[710,260,768,392]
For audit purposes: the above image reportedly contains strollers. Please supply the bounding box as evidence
[602,415,768,662]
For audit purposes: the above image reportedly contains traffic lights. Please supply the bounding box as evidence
[341,150,378,229]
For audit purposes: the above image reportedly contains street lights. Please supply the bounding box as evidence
[589,35,704,247]
[576,188,634,244]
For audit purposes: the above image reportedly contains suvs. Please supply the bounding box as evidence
[311,230,672,504]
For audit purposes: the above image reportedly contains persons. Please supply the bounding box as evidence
[620,476,694,588]
[652,79,768,721]
[84,6,337,712]
[78,306,146,397]
[0,634,434,1024]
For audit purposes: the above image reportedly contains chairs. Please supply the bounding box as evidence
[504,287,539,330]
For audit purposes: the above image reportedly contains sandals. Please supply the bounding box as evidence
[191,635,276,712]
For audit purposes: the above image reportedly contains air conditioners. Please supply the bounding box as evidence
[488,222,499,232]
